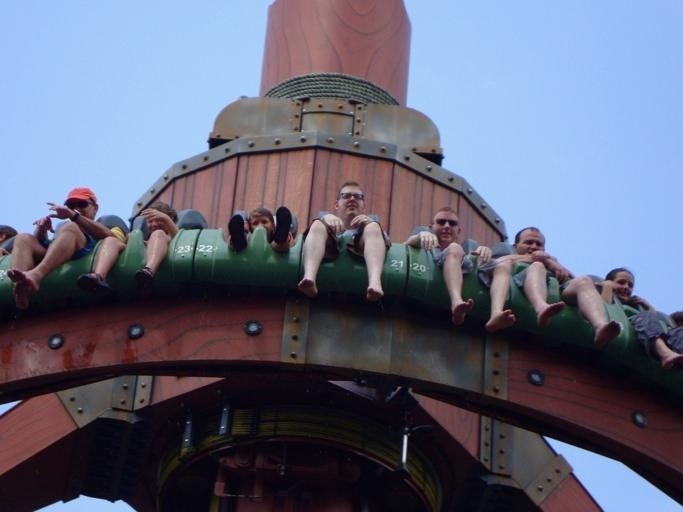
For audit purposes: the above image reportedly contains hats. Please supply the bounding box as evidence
[64,186,98,206]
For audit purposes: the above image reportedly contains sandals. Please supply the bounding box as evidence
[77,273,112,297]
[134,267,152,293]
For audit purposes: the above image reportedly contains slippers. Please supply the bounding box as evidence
[272,206,292,244]
[228,214,247,249]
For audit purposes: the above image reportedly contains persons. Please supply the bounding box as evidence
[0,187,127,310]
[76,201,178,295]
[594,267,683,370]
[403,207,516,333]
[512,226,621,346]
[297,181,392,302]
[228,206,296,253]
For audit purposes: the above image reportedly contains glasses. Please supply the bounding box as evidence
[340,193,363,199]
[436,218,459,225]
[68,202,94,209]
